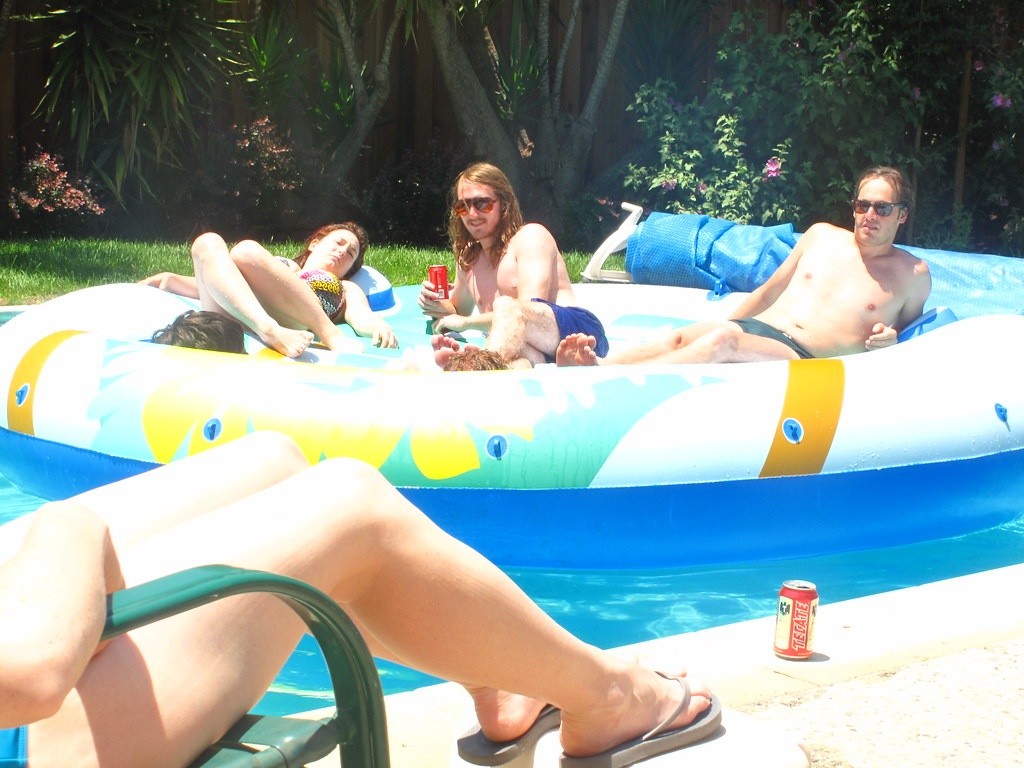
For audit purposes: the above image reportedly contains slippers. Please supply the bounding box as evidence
[561,670,722,768]
[458,703,561,766]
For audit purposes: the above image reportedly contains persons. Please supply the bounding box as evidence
[0,437,725,768]
[418,162,609,373]
[134,222,399,358]
[556,166,932,367]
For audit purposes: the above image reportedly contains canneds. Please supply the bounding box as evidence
[774,580,820,660]
[428,265,449,302]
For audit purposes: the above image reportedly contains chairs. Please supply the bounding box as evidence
[0,564,392,768]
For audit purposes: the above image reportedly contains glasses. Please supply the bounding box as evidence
[453,195,499,216]
[851,199,906,217]
